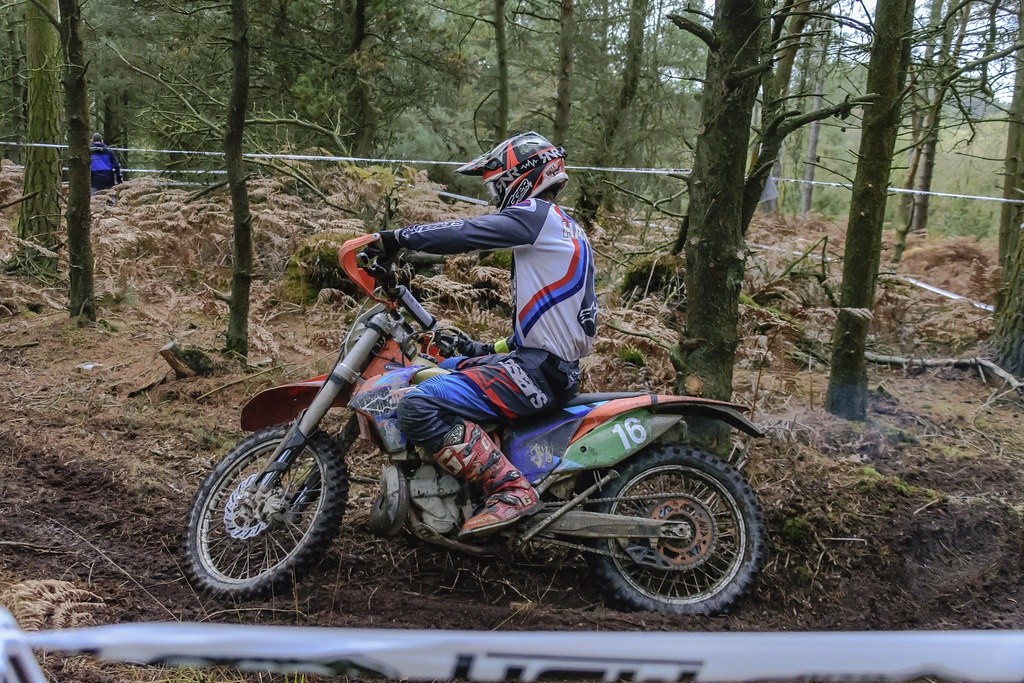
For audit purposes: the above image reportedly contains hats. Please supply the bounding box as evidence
[91,132,103,144]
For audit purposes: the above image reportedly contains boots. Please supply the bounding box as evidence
[433,417,544,541]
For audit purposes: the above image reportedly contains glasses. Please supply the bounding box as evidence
[485,182,500,204]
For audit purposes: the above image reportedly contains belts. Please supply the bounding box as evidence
[545,354,580,371]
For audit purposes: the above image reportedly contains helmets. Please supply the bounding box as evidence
[454,131,568,210]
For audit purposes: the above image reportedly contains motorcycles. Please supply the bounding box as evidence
[182,231,769,619]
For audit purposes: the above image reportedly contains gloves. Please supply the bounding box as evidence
[363,230,400,270]
[445,328,489,356]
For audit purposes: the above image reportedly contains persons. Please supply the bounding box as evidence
[361,131,603,541]
[90,132,123,196]
